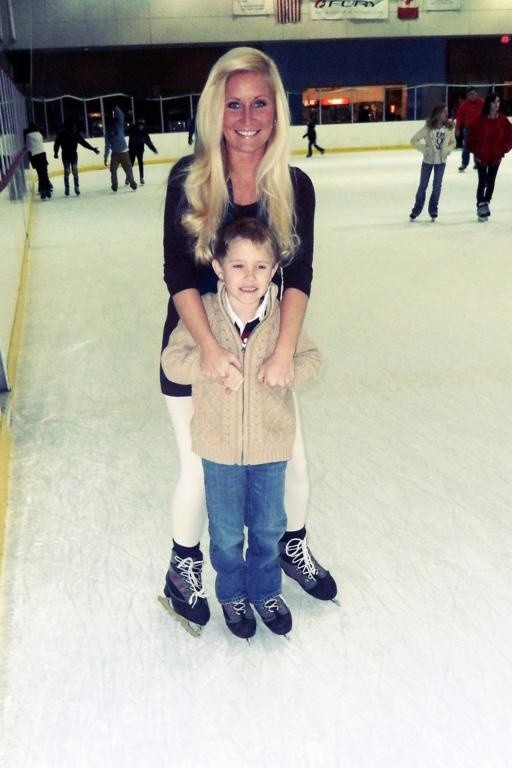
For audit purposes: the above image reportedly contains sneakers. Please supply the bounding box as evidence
[475,199,491,213]
[410,206,418,218]
[162,548,214,627]
[220,597,256,642]
[279,541,339,602]
[251,594,300,637]
[425,205,436,214]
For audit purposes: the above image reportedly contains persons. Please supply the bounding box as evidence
[302,120,326,158]
[160,220,325,644]
[455,90,489,172]
[54,119,102,195]
[28,125,53,201]
[103,105,138,193]
[124,121,159,185]
[160,46,341,638]
[466,93,512,225]
[409,106,457,221]
